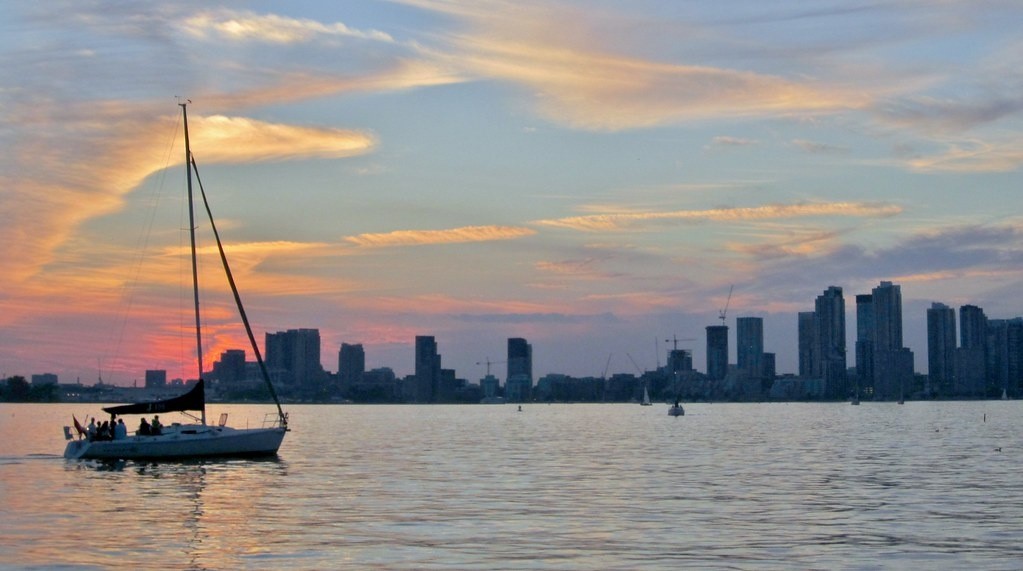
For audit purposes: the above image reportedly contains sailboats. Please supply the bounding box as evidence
[63,99,291,460]
[852,383,859,405]
[640,386,653,405]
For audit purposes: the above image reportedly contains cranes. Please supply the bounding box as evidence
[477,357,508,376]
[665,334,700,349]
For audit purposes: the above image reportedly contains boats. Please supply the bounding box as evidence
[668,394,684,417]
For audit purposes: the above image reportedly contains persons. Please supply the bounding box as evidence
[87,415,164,442]
